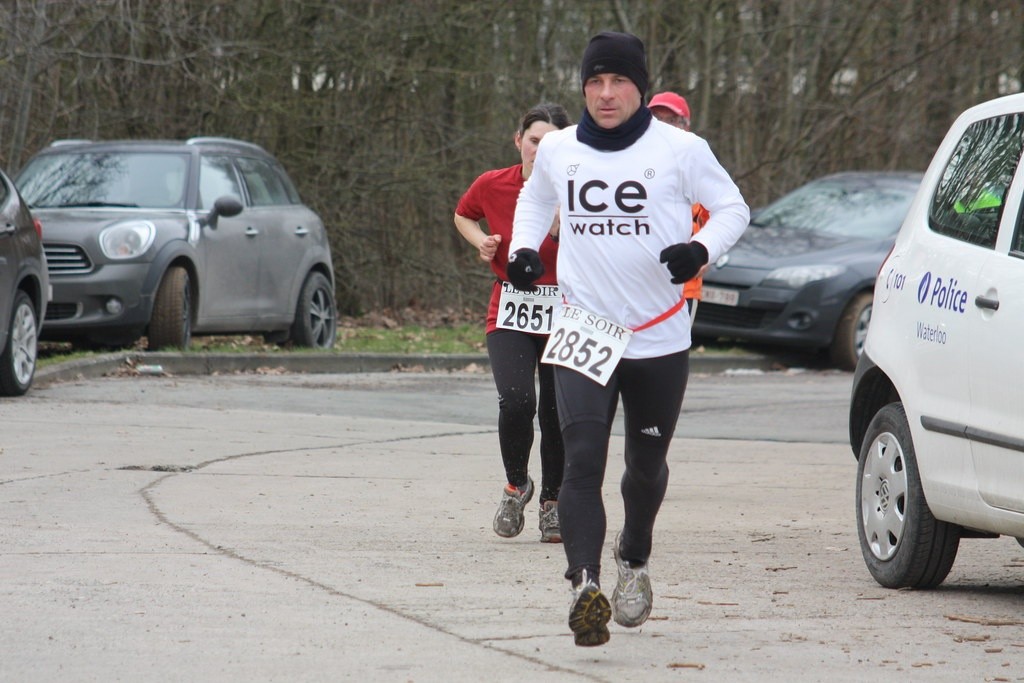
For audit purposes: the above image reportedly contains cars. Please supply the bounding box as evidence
[685,167,929,372]
[8,134,338,354]
[0,166,53,399]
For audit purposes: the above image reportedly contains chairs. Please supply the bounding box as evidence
[952,212,984,236]
[140,170,183,206]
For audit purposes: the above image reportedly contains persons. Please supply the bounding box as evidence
[454,103,565,542]
[646,92,709,328]
[506,31,750,647]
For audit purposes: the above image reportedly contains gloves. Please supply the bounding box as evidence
[506,248,546,292]
[660,240,710,285]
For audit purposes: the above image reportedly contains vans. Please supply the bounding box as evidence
[846,91,1024,596]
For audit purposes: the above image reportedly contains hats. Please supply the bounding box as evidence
[581,32,648,98]
[646,92,690,128]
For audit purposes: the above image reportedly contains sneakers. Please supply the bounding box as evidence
[568,569,612,647]
[538,493,563,543]
[492,475,535,538]
[610,529,653,627]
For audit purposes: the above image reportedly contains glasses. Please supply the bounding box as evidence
[655,115,686,127]
